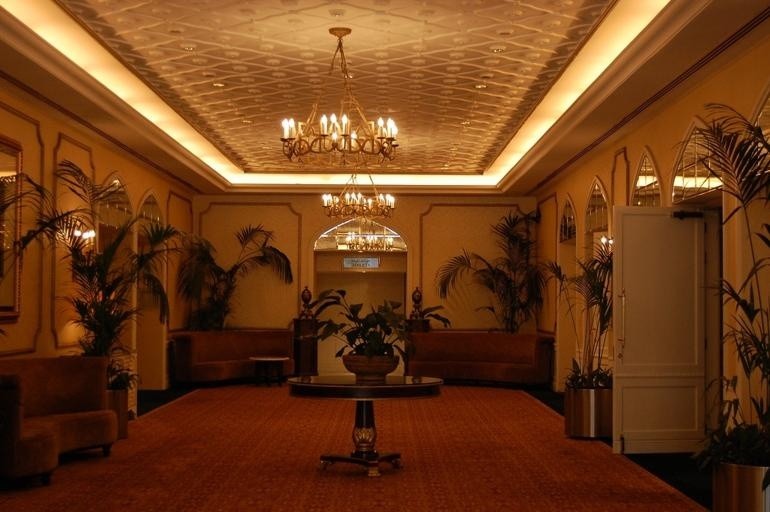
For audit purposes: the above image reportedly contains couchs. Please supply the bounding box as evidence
[404,328,555,384]
[175,329,297,386]
[0,351,124,492]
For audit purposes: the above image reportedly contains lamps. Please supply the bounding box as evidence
[279,23,402,257]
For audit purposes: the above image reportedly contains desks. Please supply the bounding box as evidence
[288,375,444,476]
[249,355,290,387]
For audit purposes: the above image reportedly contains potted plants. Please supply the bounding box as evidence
[303,283,454,384]
[54,153,178,442]
[673,82,770,512]
[540,235,619,444]
